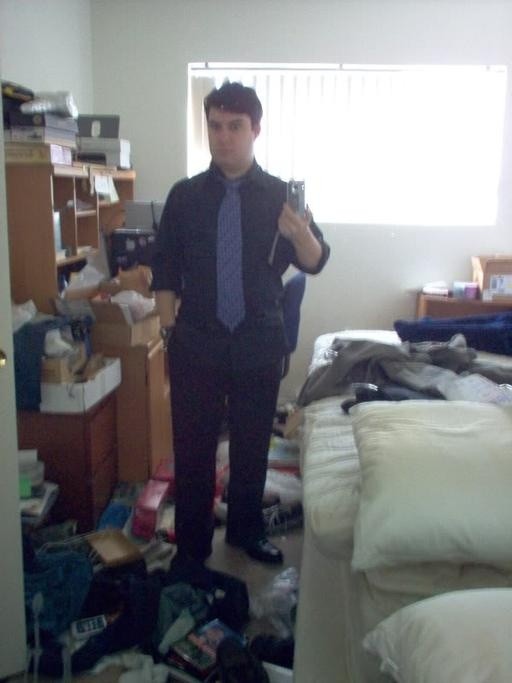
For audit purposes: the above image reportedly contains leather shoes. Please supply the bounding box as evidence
[226,532,282,562]
[172,545,212,564]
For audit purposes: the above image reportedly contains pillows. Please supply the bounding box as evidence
[356,587,512,681]
[344,393,512,579]
[389,308,511,358]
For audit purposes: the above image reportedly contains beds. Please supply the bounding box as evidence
[285,320,512,681]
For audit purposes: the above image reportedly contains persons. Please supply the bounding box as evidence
[149,76,331,579]
[195,625,227,645]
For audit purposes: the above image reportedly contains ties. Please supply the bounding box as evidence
[217,182,246,333]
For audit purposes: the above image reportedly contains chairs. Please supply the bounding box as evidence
[279,268,306,385]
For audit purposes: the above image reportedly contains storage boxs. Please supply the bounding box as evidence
[33,353,124,416]
[74,133,133,168]
[7,111,77,149]
[4,140,73,164]
[37,338,92,389]
[148,288,177,337]
[469,250,511,306]
[125,196,169,230]
[14,312,95,411]
[62,284,165,347]
[130,478,171,542]
[78,112,124,137]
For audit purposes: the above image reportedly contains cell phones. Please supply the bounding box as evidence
[286,181,305,218]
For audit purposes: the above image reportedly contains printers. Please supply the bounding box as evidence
[74,115,130,170]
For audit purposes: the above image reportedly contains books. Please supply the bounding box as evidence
[46,527,144,570]
[171,614,245,671]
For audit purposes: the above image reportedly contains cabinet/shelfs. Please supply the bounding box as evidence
[12,387,118,538]
[3,158,137,317]
[94,330,177,490]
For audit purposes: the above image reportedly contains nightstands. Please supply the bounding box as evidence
[412,288,512,327]
